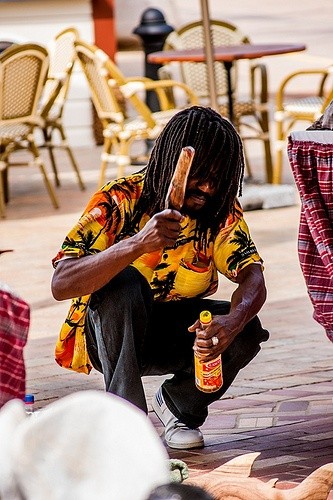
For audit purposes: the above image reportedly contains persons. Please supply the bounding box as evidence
[52,106,270,448]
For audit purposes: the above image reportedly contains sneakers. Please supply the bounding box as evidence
[152,386,205,449]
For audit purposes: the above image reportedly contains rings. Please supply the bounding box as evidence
[211,336,219,346]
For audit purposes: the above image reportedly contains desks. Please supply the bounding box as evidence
[147,43,307,121]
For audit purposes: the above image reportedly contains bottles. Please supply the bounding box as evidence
[193,310,224,393]
[23,394,35,415]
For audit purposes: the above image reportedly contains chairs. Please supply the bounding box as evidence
[158,19,273,187]
[276,65,333,184]
[1,26,79,222]
[73,40,198,188]
